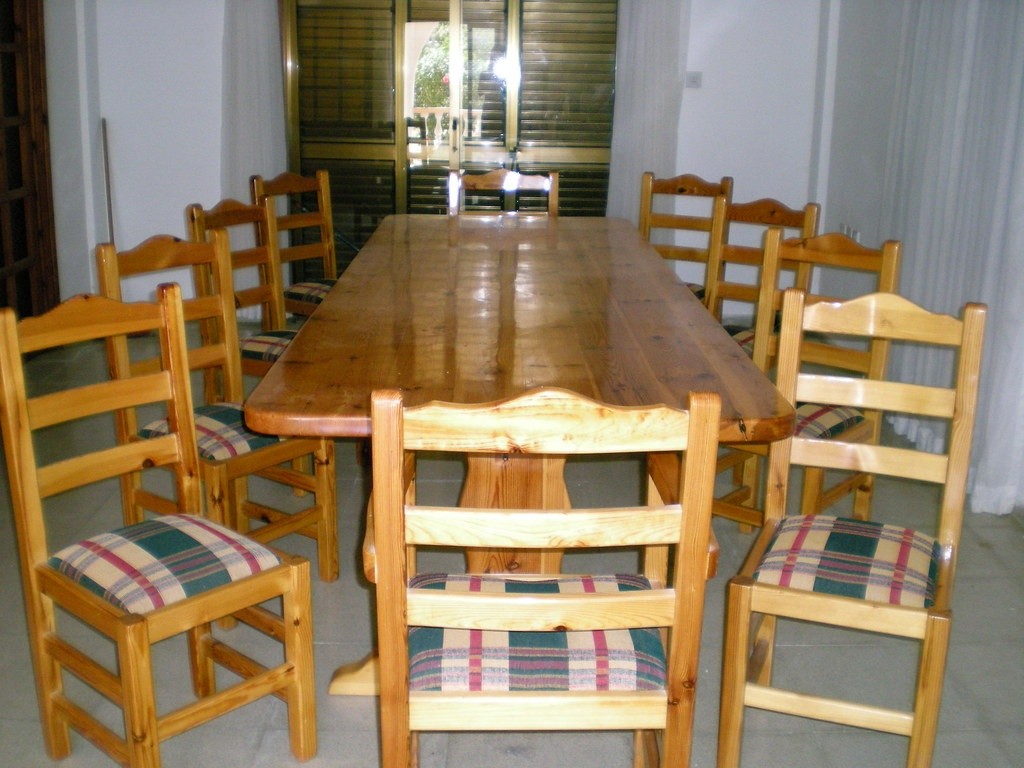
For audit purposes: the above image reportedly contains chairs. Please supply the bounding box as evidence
[95,227,337,583]
[250,169,338,328]
[362,382,723,768]
[705,194,820,381]
[184,193,309,498]
[682,227,900,528]
[0,280,315,768]
[718,290,988,768]
[447,168,559,215]
[640,171,734,312]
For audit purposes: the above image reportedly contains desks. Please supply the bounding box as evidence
[243,216,798,694]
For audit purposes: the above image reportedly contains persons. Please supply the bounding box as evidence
[477,44,506,142]
